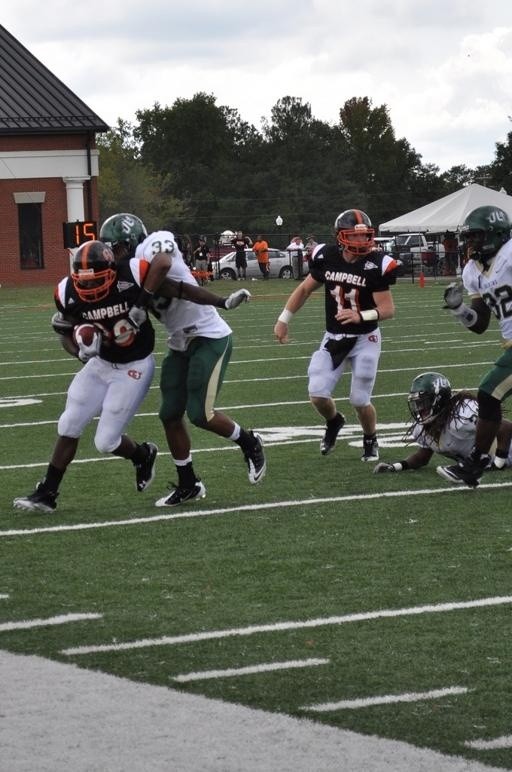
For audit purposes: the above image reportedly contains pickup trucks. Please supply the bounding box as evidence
[389,233,446,264]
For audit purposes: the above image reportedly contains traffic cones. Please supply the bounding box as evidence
[419,271,425,287]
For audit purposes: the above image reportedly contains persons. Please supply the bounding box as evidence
[286,237,304,280]
[192,235,210,272]
[230,229,253,281]
[425,246,440,267]
[436,206,512,488]
[98,213,267,508]
[9,239,252,514]
[253,235,270,281]
[273,209,406,463]
[373,372,512,476]
[443,231,459,275]
[305,236,318,270]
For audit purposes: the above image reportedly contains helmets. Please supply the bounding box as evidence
[408,371,452,426]
[72,240,116,304]
[100,213,148,261]
[458,205,510,262]
[334,208,374,257]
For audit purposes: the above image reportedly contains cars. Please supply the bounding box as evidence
[211,247,308,280]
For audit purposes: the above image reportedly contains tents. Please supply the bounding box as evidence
[379,183,512,234]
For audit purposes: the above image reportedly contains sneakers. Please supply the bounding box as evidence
[437,447,489,486]
[244,431,266,484]
[361,432,380,462]
[320,412,346,454]
[154,480,206,506]
[13,481,60,514]
[136,442,158,492]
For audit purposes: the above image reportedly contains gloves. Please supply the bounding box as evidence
[125,304,148,335]
[445,282,465,310]
[76,331,102,363]
[220,287,251,310]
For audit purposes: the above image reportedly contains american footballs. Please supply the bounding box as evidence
[73,322,100,352]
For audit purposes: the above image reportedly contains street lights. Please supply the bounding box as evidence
[276,215,284,249]
